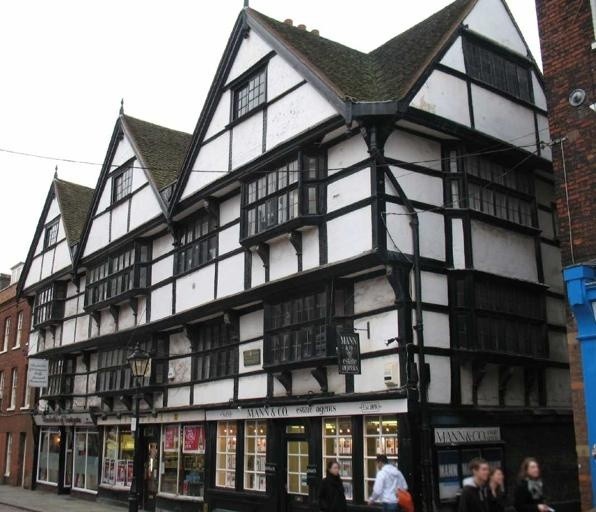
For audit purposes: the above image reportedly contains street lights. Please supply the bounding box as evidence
[126,339,153,512]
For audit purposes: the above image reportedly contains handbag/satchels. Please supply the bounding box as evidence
[394,486,418,512]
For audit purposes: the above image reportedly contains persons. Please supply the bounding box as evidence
[316,460,348,511]
[366,453,409,511]
[508,456,554,511]
[486,465,509,512]
[459,457,493,511]
